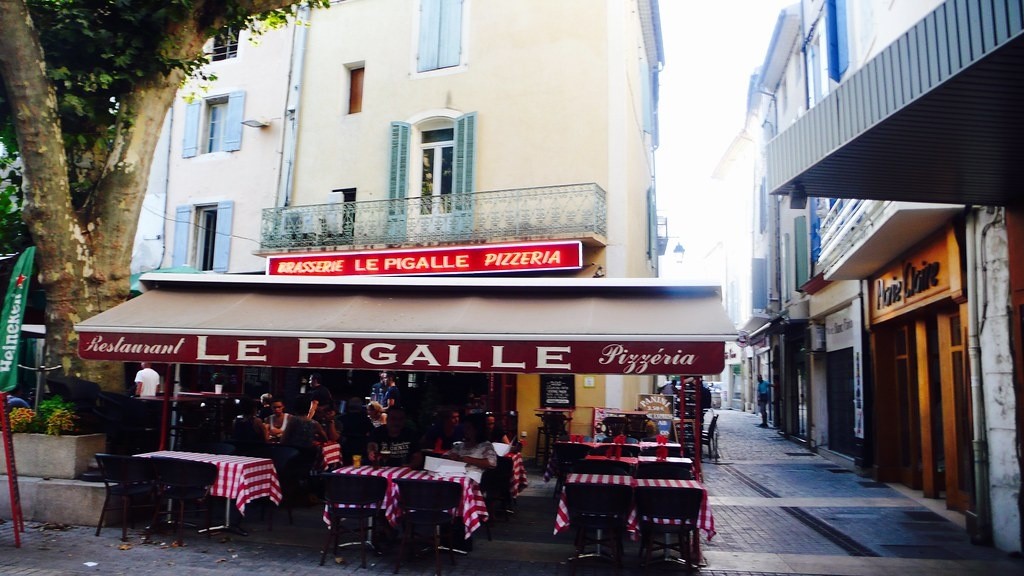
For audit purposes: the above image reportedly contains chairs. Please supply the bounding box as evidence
[566,483,632,576]
[632,486,702,576]
[100,391,147,442]
[319,473,388,568]
[700,414,718,460]
[392,478,463,576]
[146,456,218,546]
[94,452,153,542]
[48,375,101,412]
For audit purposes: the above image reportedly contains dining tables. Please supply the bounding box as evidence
[136,396,208,449]
[557,435,693,479]
[179,391,246,439]
[424,445,525,513]
[268,435,340,493]
[331,466,476,560]
[133,450,273,534]
[565,474,707,567]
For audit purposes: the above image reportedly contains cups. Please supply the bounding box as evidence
[353,455,362,469]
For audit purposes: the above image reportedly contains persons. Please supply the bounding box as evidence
[370,372,388,407]
[756,373,775,429]
[482,413,516,514]
[256,393,274,423]
[436,417,497,554]
[6,384,30,412]
[661,379,712,409]
[305,373,333,420]
[134,362,161,397]
[380,371,400,413]
[317,401,343,441]
[368,405,424,470]
[419,408,467,451]
[335,396,376,451]
[226,397,270,458]
[264,401,295,441]
[366,401,387,428]
[280,396,328,504]
